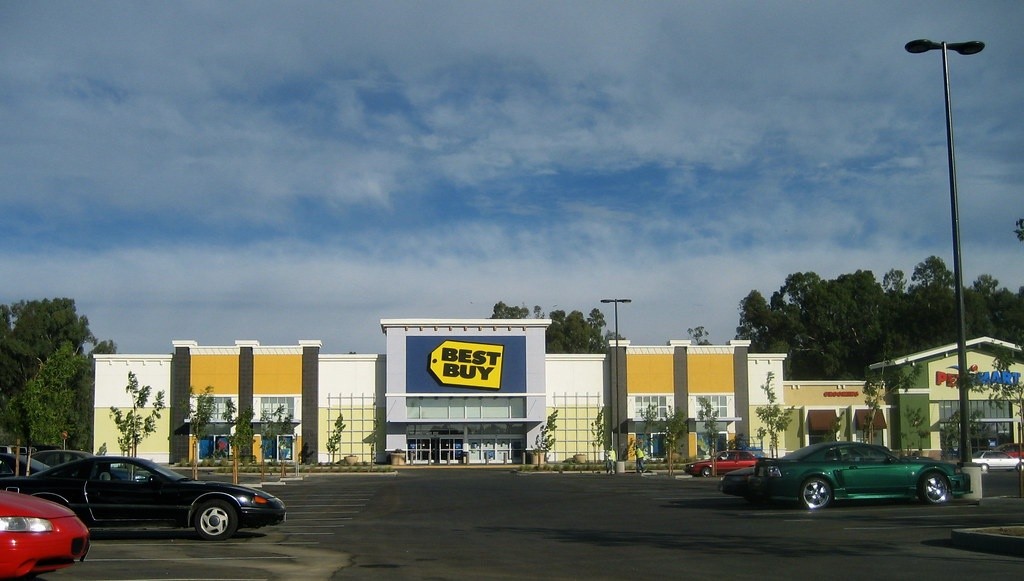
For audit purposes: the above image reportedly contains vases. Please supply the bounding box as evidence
[345,456,358,465]
[531,453,545,465]
[573,455,587,463]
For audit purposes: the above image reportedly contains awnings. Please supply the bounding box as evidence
[808,410,839,431]
[855,409,888,430]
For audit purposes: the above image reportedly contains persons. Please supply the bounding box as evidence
[217,439,227,458]
[729,439,746,450]
[604,446,616,474]
[301,442,309,463]
[635,444,645,473]
[99,443,107,455]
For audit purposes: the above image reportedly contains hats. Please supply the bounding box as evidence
[636,445,640,449]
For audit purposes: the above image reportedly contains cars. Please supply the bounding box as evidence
[753,442,973,510]
[948,451,1024,471]
[717,466,773,502]
[27,450,94,469]
[0,456,286,541]
[0,490,90,580]
[990,443,1024,459]
[0,454,50,477]
[686,451,760,477]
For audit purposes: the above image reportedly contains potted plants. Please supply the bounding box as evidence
[390,448,406,465]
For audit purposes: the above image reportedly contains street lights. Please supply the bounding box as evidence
[905,39,987,503]
[601,299,632,473]
